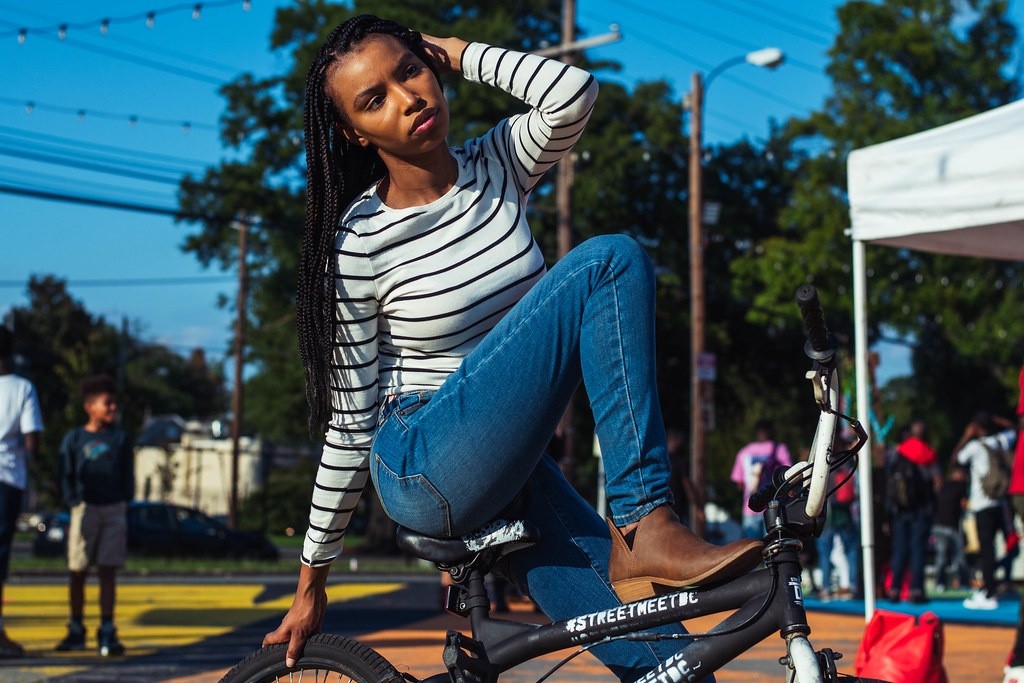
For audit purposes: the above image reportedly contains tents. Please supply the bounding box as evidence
[848,98,1024,628]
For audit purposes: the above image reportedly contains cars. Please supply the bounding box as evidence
[32,500,283,564]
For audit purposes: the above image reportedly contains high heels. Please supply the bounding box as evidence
[606,503,765,605]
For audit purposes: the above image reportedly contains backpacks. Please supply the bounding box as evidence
[980,440,1009,499]
[890,458,927,511]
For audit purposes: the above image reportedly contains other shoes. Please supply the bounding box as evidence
[815,590,832,599]
[962,596,999,610]
[0,630,24,658]
[55,622,85,651]
[910,593,923,602]
[839,588,852,600]
[98,627,125,655]
[888,594,899,602]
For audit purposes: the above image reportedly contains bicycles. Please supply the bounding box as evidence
[223,283,897,683]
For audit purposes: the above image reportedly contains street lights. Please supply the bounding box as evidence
[688,46,789,544]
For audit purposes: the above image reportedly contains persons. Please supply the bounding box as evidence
[0,328,138,658]
[262,14,764,682]
[668,367,1024,683]
